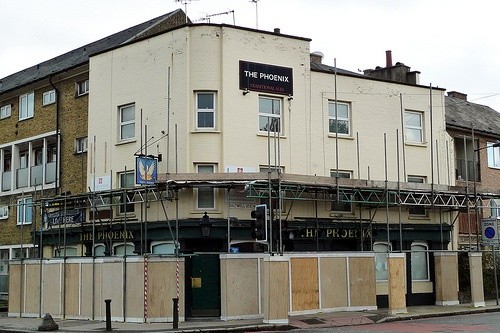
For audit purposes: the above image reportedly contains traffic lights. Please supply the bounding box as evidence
[250,204,268,242]
